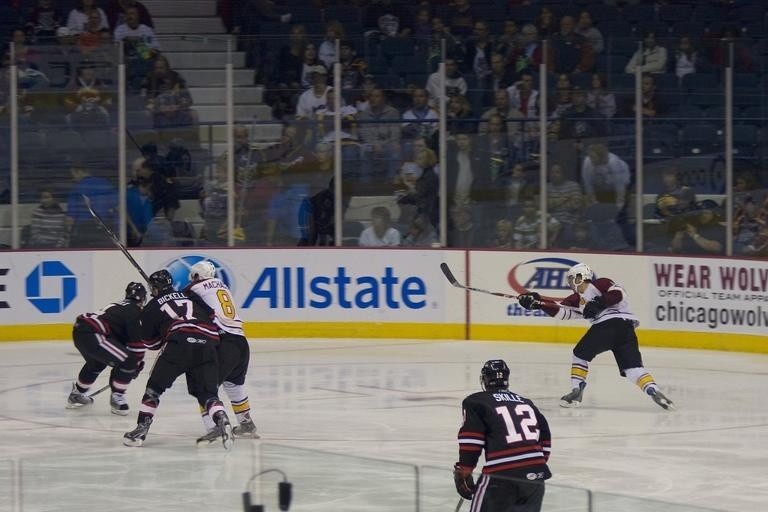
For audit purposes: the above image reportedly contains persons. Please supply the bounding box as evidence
[67,282,147,414]
[0,0,768,256]
[181,260,257,447]
[517,263,674,410]
[453,359,553,512]
[121,270,235,450]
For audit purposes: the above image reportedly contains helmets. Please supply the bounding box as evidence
[126,282,146,301]
[568,264,594,283]
[479,360,510,390]
[188,262,216,281]
[148,270,172,293]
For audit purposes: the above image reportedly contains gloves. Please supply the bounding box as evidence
[518,292,542,310]
[583,298,606,319]
[453,462,477,501]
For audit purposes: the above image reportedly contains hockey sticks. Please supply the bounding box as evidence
[441,261,585,317]
[82,193,151,285]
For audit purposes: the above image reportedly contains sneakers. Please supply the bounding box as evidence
[233,414,255,435]
[647,387,673,408]
[196,430,222,442]
[216,412,234,450]
[110,393,127,410]
[124,417,153,439]
[67,393,94,405]
[562,383,586,403]
